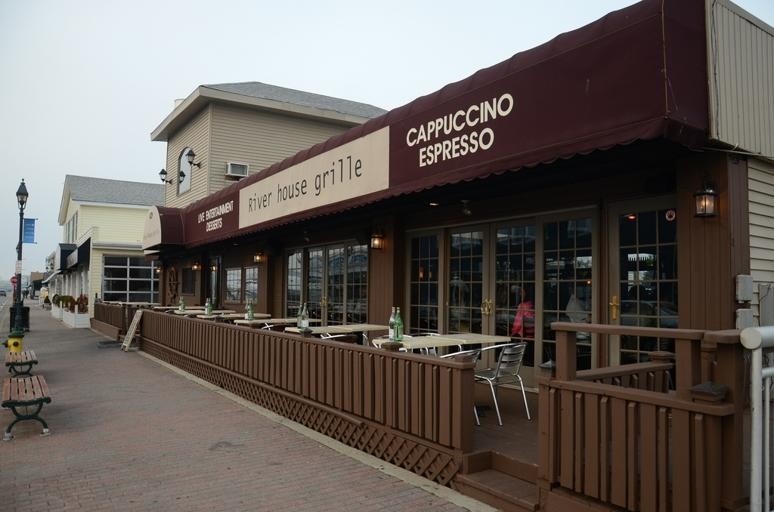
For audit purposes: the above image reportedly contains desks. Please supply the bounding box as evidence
[9,179,29,337]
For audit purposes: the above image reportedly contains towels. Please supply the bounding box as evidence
[9,307,30,332]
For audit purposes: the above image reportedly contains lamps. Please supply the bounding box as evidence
[320,332,531,426]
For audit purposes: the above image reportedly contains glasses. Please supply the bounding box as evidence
[369,233,385,251]
[695,188,718,220]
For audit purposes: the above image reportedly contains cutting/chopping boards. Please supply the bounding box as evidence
[2,328,24,352]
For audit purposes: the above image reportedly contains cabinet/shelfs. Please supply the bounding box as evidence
[297,303,310,330]
[204,297,212,316]
[388,306,404,342]
[179,296,185,312]
[244,298,254,320]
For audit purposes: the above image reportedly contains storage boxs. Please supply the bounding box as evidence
[0,349,51,441]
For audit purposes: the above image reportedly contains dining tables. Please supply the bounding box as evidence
[49,293,90,330]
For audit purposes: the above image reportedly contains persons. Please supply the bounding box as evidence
[565,293,592,340]
[22,289,29,299]
[509,299,535,344]
